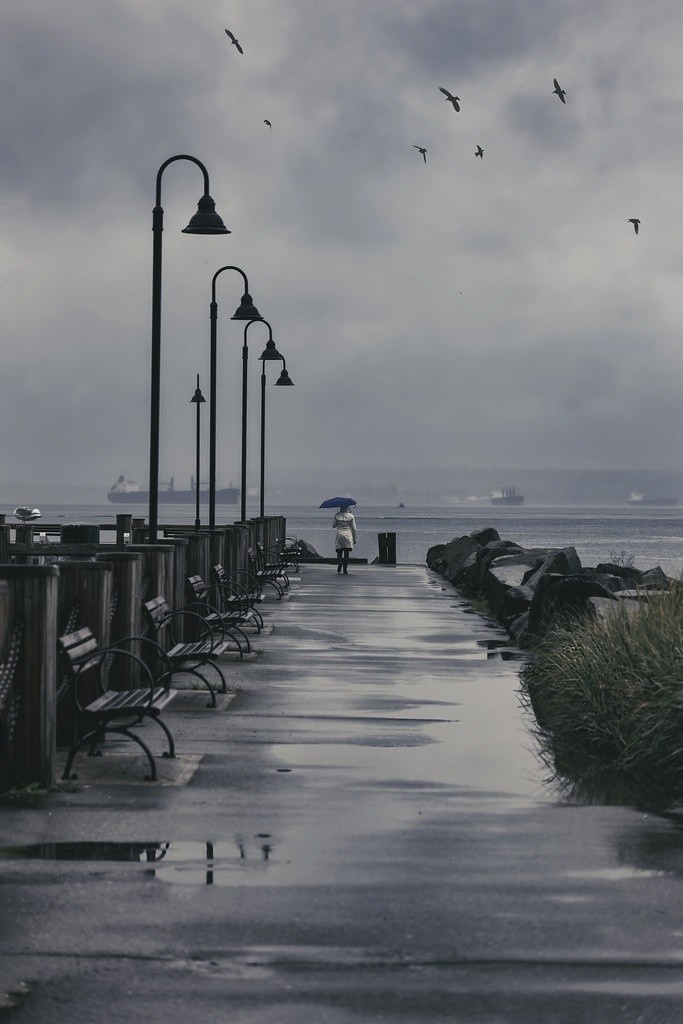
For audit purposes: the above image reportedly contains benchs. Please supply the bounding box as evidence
[59,627,178,782]
[187,574,251,660]
[144,596,228,707]
[213,564,265,634]
[247,548,283,600]
[276,534,303,573]
[257,542,289,587]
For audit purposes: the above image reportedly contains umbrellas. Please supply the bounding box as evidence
[319,498,356,508]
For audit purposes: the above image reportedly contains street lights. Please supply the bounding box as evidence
[188,373,208,530]
[259,355,296,517]
[240,319,284,522]
[146,153,233,545]
[205,264,265,530]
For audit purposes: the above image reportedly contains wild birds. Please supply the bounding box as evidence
[475,145,484,158]
[552,78,567,103]
[413,144,426,162]
[440,87,460,112]
[627,218,641,235]
[225,30,243,54]
[264,119,272,129]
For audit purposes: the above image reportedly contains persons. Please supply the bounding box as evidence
[332,506,356,573]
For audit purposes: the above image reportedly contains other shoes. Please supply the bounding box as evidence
[343,567,347,573]
[338,565,341,572]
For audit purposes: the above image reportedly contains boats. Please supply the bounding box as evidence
[103,473,241,506]
[625,488,645,506]
[396,503,406,508]
[489,485,525,506]
